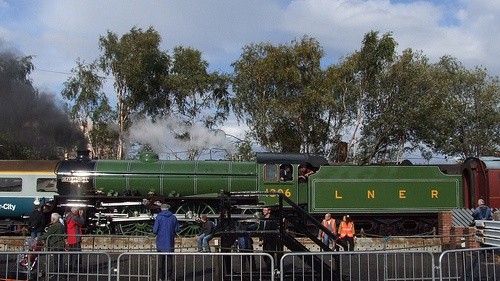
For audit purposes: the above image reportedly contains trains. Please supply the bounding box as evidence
[0,154,500,234]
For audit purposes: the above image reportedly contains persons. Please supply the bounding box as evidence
[472,199,491,220]
[27,202,58,239]
[298,165,313,182]
[280,166,290,180]
[259,207,277,271]
[238,221,257,271]
[197,215,216,252]
[35,213,66,279]
[153,204,181,281]
[338,215,355,251]
[65,206,84,272]
[317,213,338,250]
